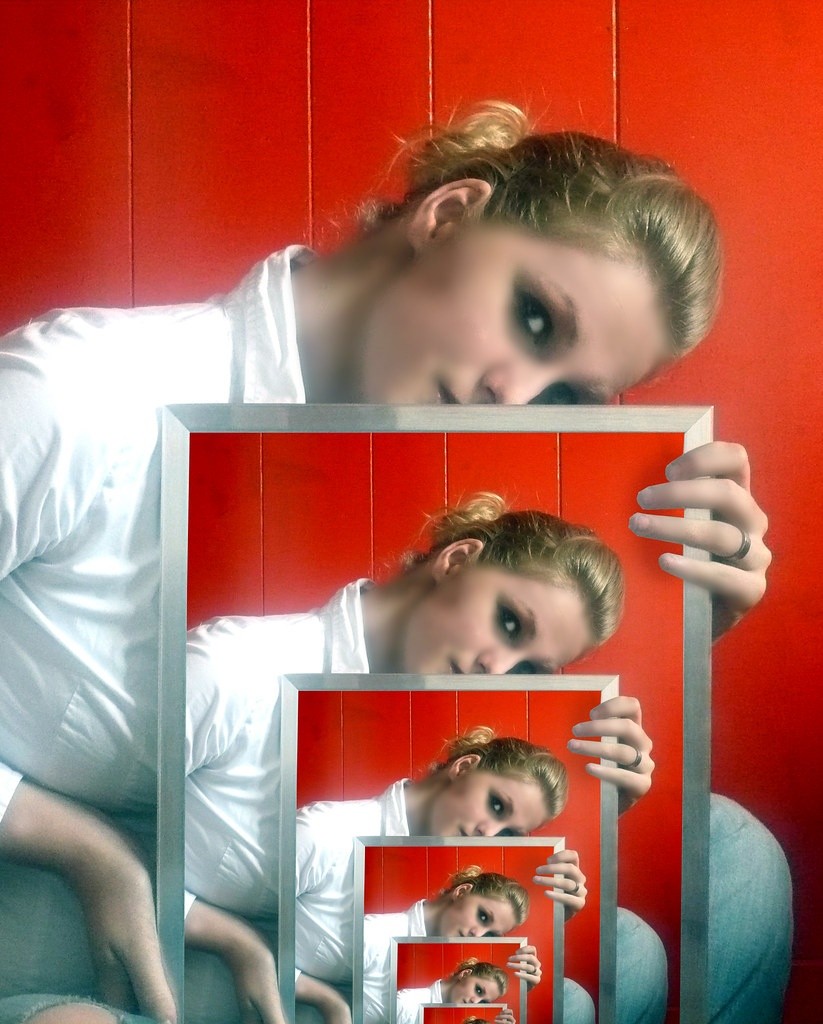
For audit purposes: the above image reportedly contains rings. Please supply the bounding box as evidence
[713,525,753,563]
[617,741,642,770]
[567,880,580,895]
[528,964,537,975]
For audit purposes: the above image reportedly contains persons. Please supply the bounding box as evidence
[0,102,793,1024]
[188,493,668,1024]
